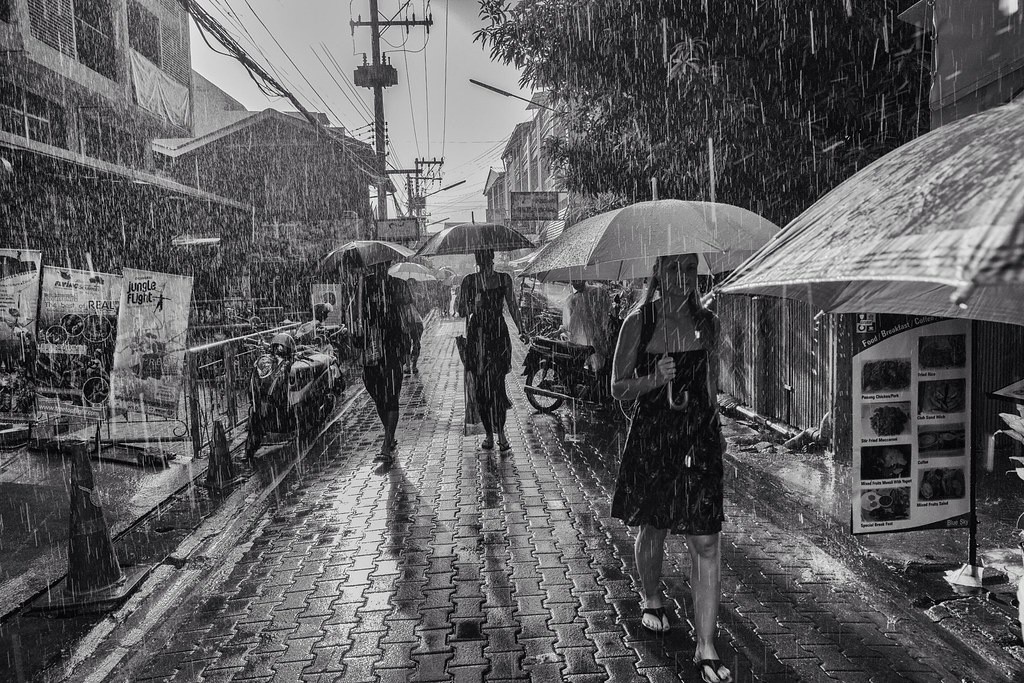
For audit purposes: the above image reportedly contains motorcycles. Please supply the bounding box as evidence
[242,303,348,460]
[516,285,636,423]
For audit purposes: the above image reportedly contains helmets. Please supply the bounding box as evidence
[315,303,330,323]
[270,333,296,352]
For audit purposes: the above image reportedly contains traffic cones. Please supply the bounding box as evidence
[30,439,151,614]
[196,419,246,489]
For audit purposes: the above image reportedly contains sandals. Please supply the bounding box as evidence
[497,440,510,452]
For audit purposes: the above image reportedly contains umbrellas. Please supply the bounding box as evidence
[320,240,454,282]
[410,210,536,254]
[714,95,1023,329]
[521,178,787,410]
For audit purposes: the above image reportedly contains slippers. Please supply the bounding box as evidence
[381,438,397,451]
[642,598,671,631]
[482,437,494,450]
[693,656,732,683]
[373,453,395,463]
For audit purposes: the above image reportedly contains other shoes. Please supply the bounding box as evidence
[412,367,418,372]
[404,367,410,373]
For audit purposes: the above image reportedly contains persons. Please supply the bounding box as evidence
[458,250,528,450]
[568,277,613,374]
[611,253,734,683]
[348,277,459,463]
